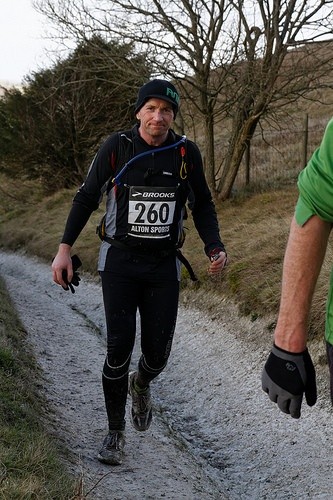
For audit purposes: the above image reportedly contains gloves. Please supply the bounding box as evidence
[52,255,82,294]
[261,342,317,419]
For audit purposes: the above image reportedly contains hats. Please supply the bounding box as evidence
[132,79,180,114]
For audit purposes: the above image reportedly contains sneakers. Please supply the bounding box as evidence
[97,431,127,465]
[128,370,153,431]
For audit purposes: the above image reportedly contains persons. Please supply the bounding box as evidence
[50,79,229,466]
[260,113,333,418]
